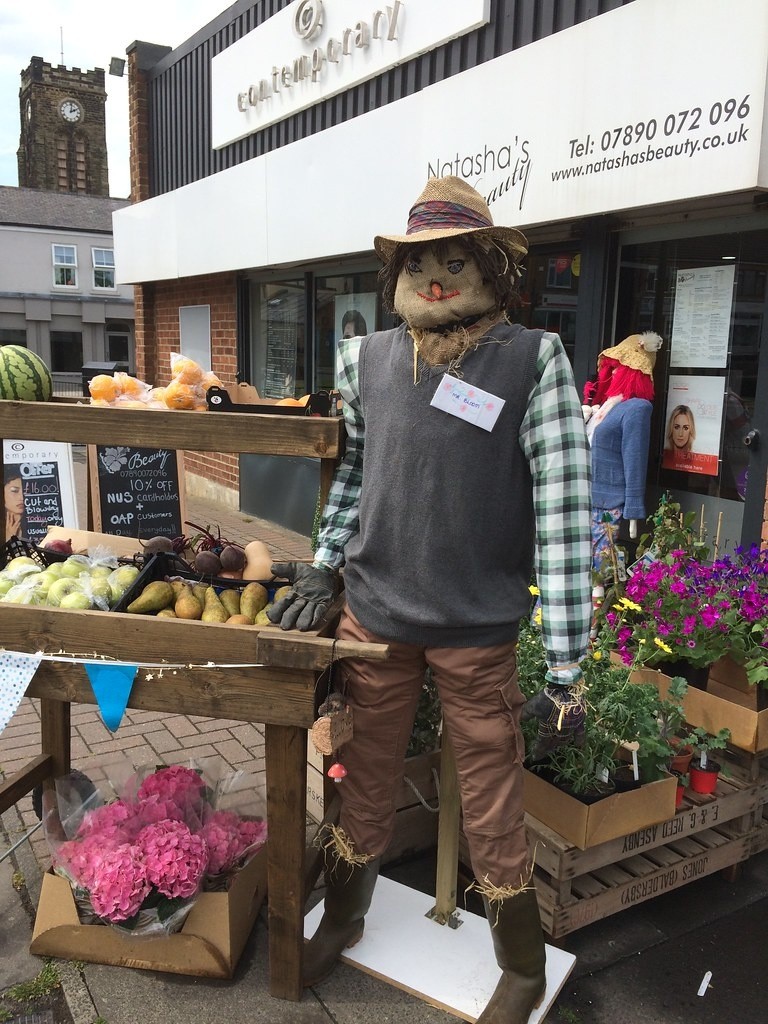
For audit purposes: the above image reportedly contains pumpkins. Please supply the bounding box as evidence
[242,540,277,581]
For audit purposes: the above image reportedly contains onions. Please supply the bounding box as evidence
[43,538,73,562]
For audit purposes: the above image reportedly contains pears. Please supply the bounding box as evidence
[125,581,294,627]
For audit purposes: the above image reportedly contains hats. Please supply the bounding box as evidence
[374,175,529,265]
[596,331,663,384]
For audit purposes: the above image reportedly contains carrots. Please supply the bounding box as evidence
[431,282,442,298]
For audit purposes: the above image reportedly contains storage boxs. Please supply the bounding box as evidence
[25,813,269,980]
[204,382,342,415]
[0,534,155,610]
[609,647,768,755]
[111,546,293,634]
[307,726,441,870]
[523,736,679,852]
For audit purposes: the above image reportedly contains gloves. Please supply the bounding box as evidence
[266,561,345,632]
[519,687,585,762]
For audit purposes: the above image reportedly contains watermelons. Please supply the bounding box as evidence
[0,345,52,402]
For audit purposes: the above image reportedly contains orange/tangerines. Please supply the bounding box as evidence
[87,361,225,412]
[274,394,310,407]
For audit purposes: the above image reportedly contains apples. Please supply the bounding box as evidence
[0,554,140,611]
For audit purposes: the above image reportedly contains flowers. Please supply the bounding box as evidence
[53,739,265,924]
[605,535,768,691]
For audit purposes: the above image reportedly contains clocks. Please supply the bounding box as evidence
[58,97,84,125]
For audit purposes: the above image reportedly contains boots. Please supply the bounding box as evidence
[300,844,380,986]
[474,873,547,1024]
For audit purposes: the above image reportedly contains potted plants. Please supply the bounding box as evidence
[536,672,731,826]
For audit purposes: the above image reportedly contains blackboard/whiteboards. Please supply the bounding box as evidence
[2,439,80,539]
[86,444,187,542]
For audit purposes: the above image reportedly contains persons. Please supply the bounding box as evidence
[530,331,664,648]
[341,310,367,340]
[1,464,24,544]
[664,405,698,471]
[266,173,593,1024]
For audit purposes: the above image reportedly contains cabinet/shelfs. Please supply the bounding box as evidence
[0,395,390,1006]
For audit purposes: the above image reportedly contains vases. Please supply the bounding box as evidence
[654,655,710,691]
